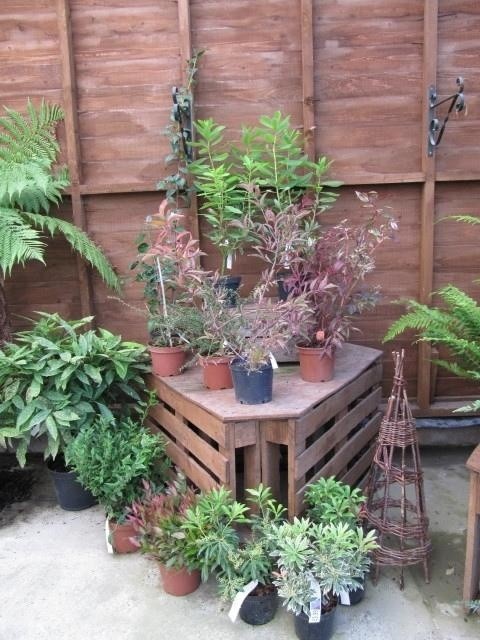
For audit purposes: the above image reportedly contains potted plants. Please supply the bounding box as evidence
[195,269,243,389]
[2,311,152,512]
[69,415,174,555]
[268,516,381,637]
[180,482,290,625]
[126,480,202,596]
[301,475,369,606]
[154,112,345,307]
[2,97,124,504]
[128,231,188,376]
[227,185,314,404]
[282,189,400,382]
